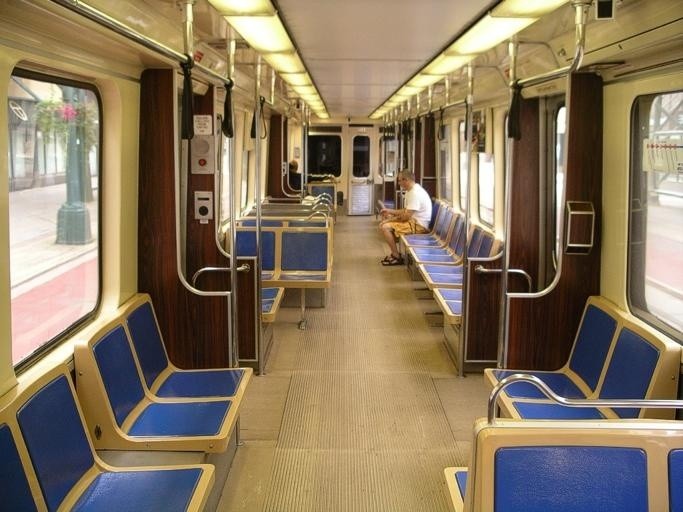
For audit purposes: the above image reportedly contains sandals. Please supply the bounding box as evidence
[381,253,403,266]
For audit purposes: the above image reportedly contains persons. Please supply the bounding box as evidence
[289,160,301,190]
[379,169,432,265]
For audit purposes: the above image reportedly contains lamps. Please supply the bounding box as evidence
[367,1,569,120]
[208,1,330,120]
